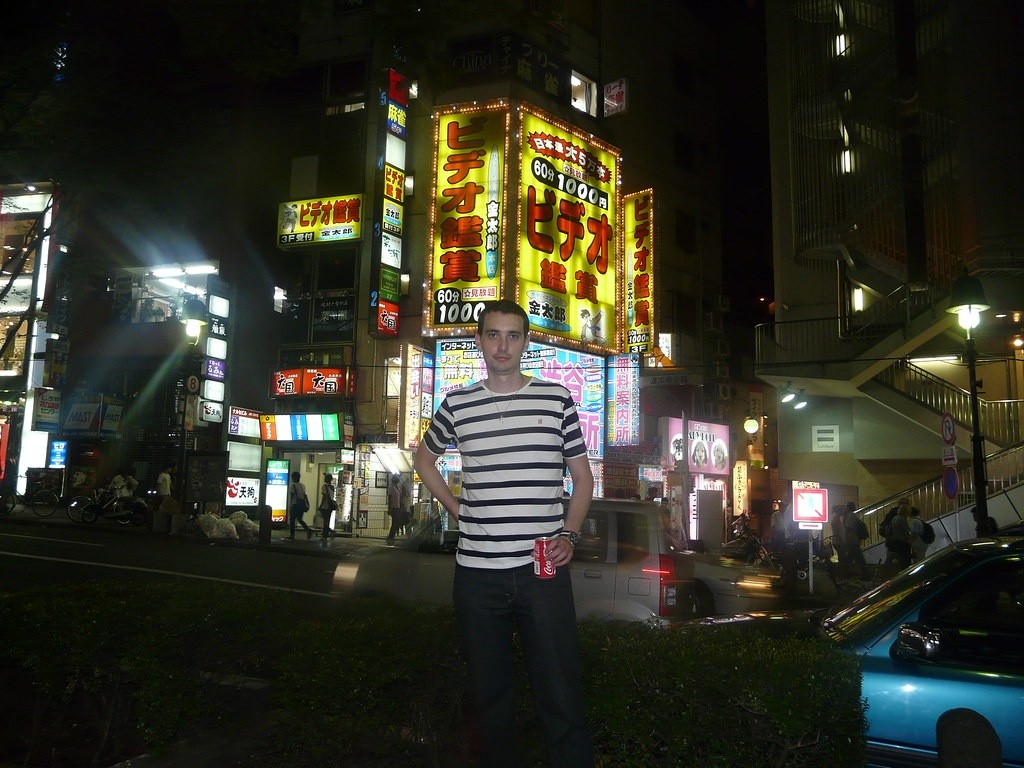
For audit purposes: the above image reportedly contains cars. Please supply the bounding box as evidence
[665,535,1024,768]
[671,545,775,618]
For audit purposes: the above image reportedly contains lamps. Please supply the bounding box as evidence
[777,382,807,410]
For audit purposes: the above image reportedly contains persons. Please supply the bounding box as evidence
[108,469,139,497]
[770,500,867,572]
[970,505,998,539]
[317,473,336,541]
[414,298,594,768]
[388,474,412,544]
[157,465,175,506]
[286,472,312,540]
[616,484,684,551]
[879,496,928,568]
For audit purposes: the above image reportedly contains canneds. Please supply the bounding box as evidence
[533,536,558,580]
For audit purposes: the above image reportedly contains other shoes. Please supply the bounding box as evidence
[320,537,327,541]
[331,531,335,540]
[307,529,312,539]
[285,537,294,541]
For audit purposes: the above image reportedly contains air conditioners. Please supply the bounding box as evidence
[703,294,730,420]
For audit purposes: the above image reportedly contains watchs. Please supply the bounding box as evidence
[559,529,581,547]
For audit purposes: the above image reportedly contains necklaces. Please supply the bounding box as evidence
[485,376,523,422]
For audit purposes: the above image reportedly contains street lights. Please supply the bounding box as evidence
[939,276,993,536]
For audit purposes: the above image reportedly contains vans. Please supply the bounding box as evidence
[335,493,696,629]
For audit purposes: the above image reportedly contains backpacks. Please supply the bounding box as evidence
[916,518,935,543]
[850,514,869,539]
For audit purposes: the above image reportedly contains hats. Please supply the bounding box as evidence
[323,473,332,481]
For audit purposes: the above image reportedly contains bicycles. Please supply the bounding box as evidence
[0,481,61,518]
[66,487,130,526]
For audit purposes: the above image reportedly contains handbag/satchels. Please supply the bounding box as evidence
[329,500,337,510]
[297,497,309,512]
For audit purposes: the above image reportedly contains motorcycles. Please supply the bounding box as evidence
[81,483,151,528]
